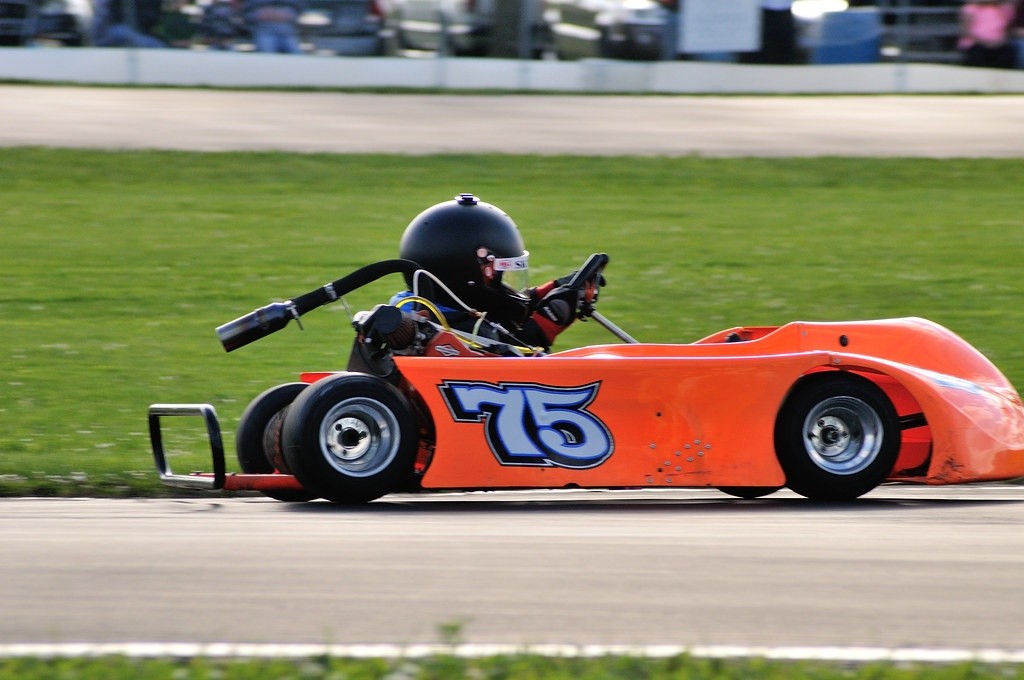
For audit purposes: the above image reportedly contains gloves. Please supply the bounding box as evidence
[538,270,607,323]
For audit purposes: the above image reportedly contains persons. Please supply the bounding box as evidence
[957,0,1020,70]
[388,193,607,357]
[24,0,303,54]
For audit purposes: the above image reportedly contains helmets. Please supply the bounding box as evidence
[399,194,527,310]
